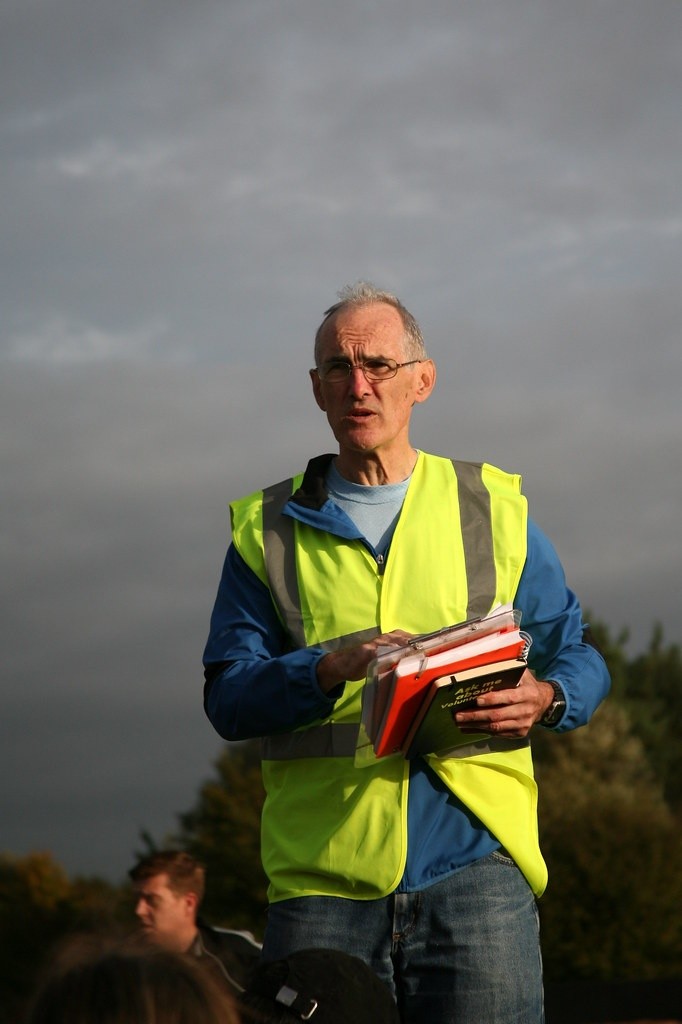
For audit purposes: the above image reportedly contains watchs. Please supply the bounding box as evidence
[538,679,567,731]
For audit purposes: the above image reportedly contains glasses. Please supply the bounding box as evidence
[313,357,422,384]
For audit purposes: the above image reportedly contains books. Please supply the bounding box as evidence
[359,604,533,763]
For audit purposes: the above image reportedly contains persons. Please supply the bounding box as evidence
[129,848,270,1023]
[28,945,244,1024]
[190,294,612,1024]
